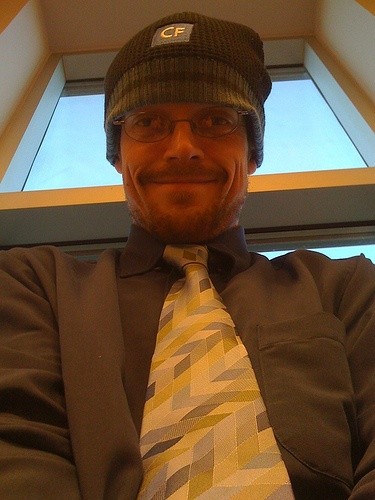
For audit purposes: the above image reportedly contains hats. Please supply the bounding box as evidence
[104,12,272,168]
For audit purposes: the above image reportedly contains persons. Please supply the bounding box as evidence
[1,10,374,499]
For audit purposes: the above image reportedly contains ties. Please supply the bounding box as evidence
[136,241,295,500]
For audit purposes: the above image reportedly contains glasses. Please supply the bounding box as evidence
[112,106,249,143]
